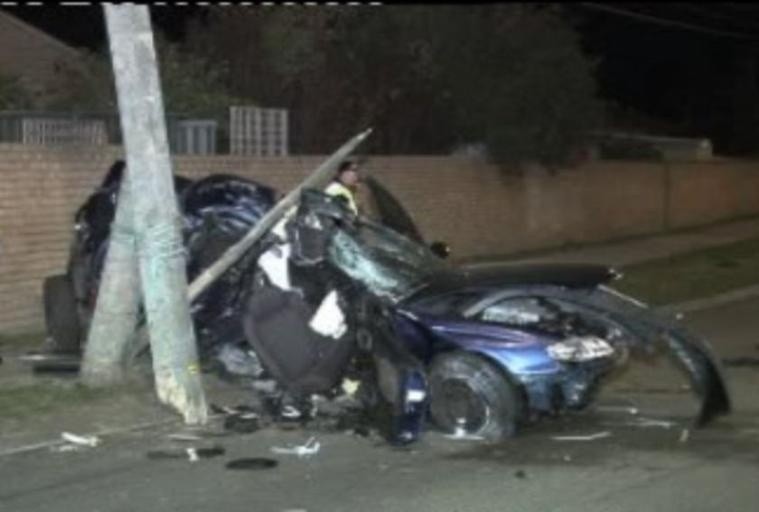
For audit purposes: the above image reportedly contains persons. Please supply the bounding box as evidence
[320,159,368,217]
